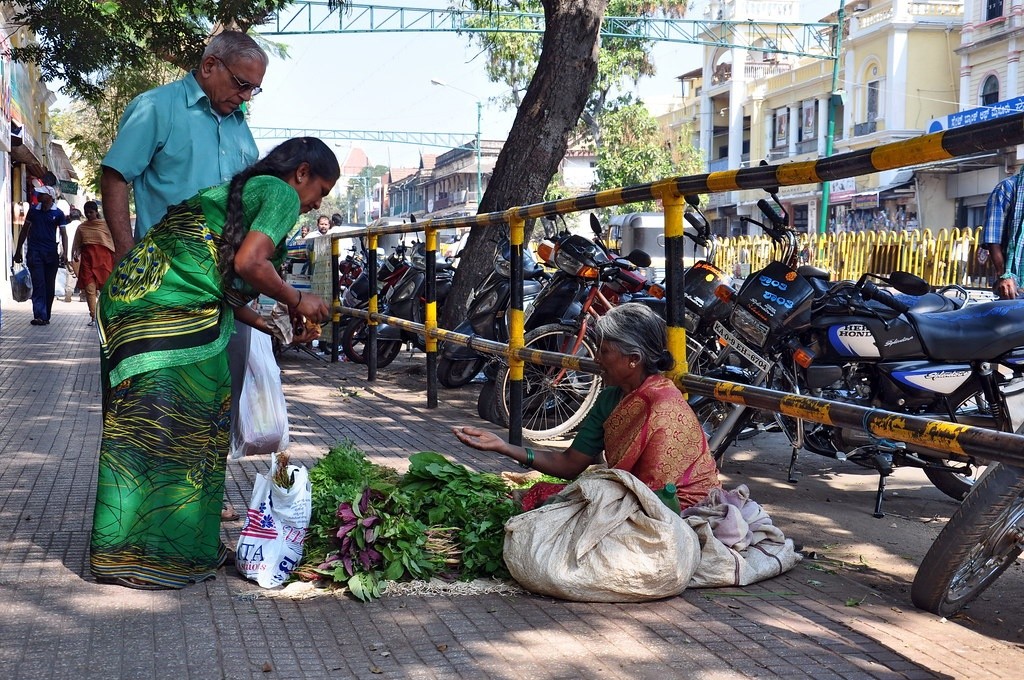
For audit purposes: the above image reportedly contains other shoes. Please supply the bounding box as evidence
[30,319,46,325]
[89,322,95,326]
[46,320,50,324]
[65,291,72,302]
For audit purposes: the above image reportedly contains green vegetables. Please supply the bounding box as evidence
[285,440,572,601]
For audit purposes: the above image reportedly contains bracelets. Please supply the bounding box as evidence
[290,288,302,309]
[518,446,535,470]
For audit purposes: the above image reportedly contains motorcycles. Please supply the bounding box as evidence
[908,380,1024,619]
[269,189,768,440]
[681,157,1024,521]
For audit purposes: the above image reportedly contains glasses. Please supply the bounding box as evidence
[213,54,263,96]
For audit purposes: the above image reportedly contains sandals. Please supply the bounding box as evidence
[221,506,240,521]
[96,576,170,590]
[223,548,236,565]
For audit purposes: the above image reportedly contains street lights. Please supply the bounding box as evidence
[430,78,483,205]
[334,143,372,222]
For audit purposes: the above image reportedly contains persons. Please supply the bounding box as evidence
[70,202,116,327]
[297,213,353,266]
[56,198,91,302]
[451,303,722,520]
[92,136,341,589]
[15,186,69,326]
[99,32,269,267]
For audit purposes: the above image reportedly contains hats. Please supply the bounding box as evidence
[34,186,55,199]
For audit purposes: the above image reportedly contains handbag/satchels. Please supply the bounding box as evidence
[10,262,33,302]
[235,452,312,589]
[229,327,289,459]
[55,263,66,296]
